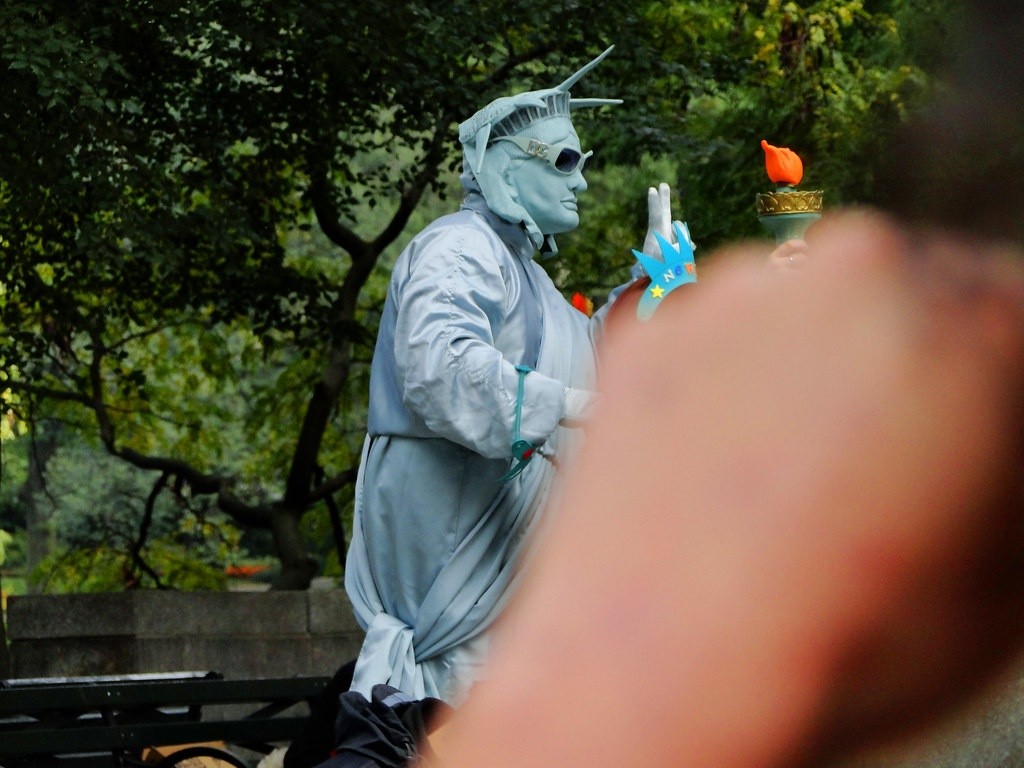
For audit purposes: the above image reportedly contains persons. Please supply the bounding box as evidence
[409,0,1024,768]
[344,42,697,768]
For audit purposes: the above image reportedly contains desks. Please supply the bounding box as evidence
[0,670,224,768]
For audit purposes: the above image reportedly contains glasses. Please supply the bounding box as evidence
[490,133,597,175]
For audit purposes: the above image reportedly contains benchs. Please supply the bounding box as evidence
[0,677,340,768]
